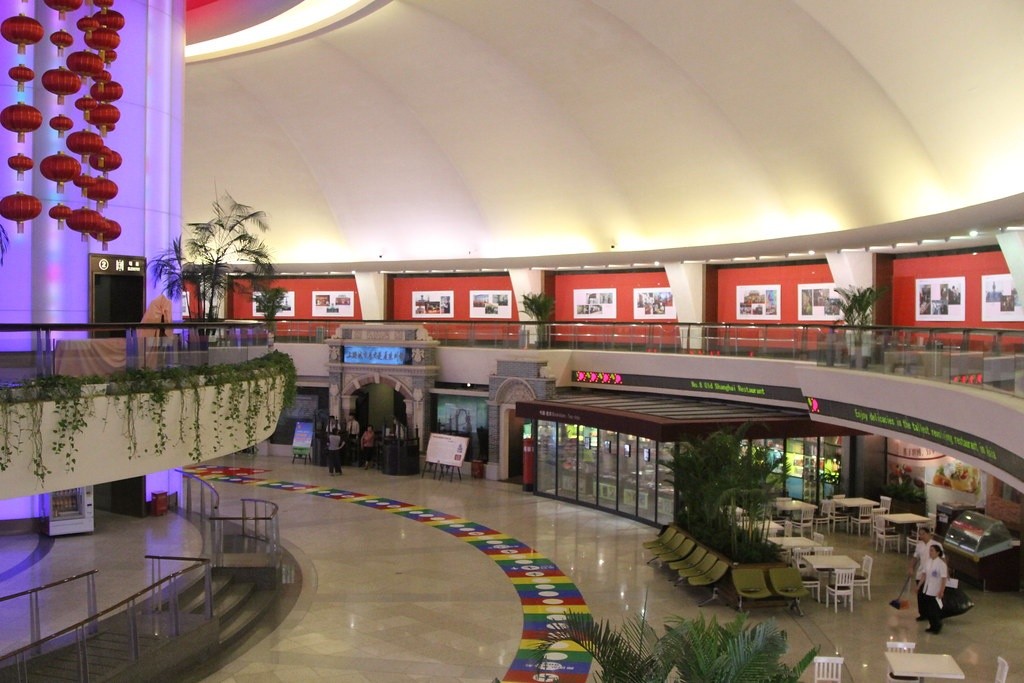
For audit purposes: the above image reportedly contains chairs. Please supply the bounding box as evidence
[995,656,1009,683]
[886,642,920,683]
[814,656,844,683]
[642,495,934,617]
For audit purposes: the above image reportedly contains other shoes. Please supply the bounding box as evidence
[328,472,341,476]
[916,616,928,621]
[364,464,368,469]
[925,623,943,634]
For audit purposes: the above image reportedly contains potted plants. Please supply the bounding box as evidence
[666,419,791,593]
[518,292,556,350]
[825,281,892,370]
[145,177,292,368]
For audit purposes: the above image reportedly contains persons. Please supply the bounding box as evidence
[346,412,359,459]
[394,418,405,438]
[907,527,947,621]
[360,425,375,470]
[326,416,345,476]
[914,545,948,634]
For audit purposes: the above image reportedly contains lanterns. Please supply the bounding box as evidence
[0,12,45,54]
[49,202,72,230]
[66,206,104,241]
[8,64,36,91]
[8,153,35,181]
[49,114,74,138]
[49,28,74,58]
[44,0,124,251]
[67,129,103,163]
[40,150,82,193]
[0,191,42,233]
[0,102,44,143]
[76,15,100,39]
[42,65,81,105]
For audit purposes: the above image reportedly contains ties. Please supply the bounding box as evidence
[350,422,353,434]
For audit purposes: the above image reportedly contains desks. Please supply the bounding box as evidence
[737,520,784,542]
[878,513,932,552]
[884,651,965,683]
[832,498,880,534]
[802,554,860,604]
[55,338,159,377]
[763,537,821,568]
[776,501,818,536]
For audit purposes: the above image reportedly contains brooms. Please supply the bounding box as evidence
[899,591,916,610]
[888,576,911,610]
